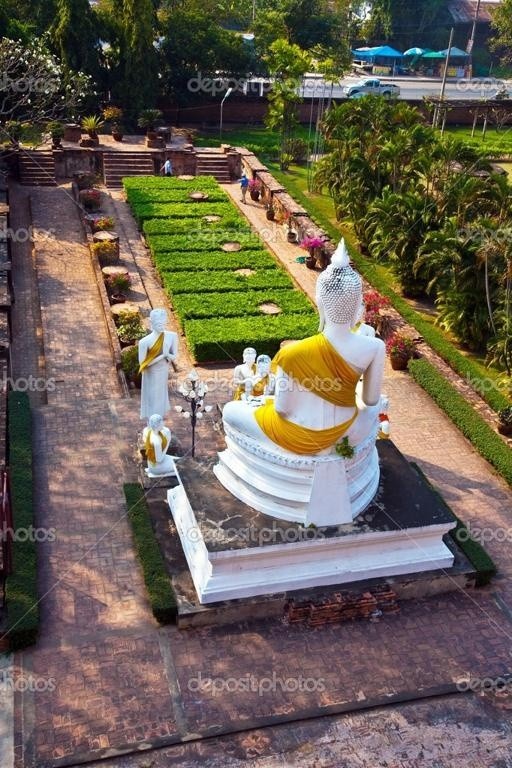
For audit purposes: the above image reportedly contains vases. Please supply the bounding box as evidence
[110,292,127,304]
[390,355,407,370]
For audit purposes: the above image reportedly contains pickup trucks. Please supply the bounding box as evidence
[342,77,402,98]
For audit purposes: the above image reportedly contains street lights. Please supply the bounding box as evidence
[219,87,233,139]
[173,366,215,457]
[249,6,255,21]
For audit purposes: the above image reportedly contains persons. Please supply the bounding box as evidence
[159,156,175,177]
[237,173,249,204]
[234,346,257,400]
[241,354,276,401]
[221,236,387,457]
[138,308,180,420]
[143,413,181,476]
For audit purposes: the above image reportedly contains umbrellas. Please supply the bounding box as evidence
[403,46,425,56]
[421,50,444,64]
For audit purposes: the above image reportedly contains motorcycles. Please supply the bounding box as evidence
[495,89,510,99]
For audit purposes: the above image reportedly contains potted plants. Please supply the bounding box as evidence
[117,307,149,389]
[45,119,65,146]
[496,403,512,434]
[80,113,105,138]
[5,120,23,144]
[102,105,124,141]
[137,107,166,140]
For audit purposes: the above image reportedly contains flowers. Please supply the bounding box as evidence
[247,176,415,357]
[72,168,132,297]
